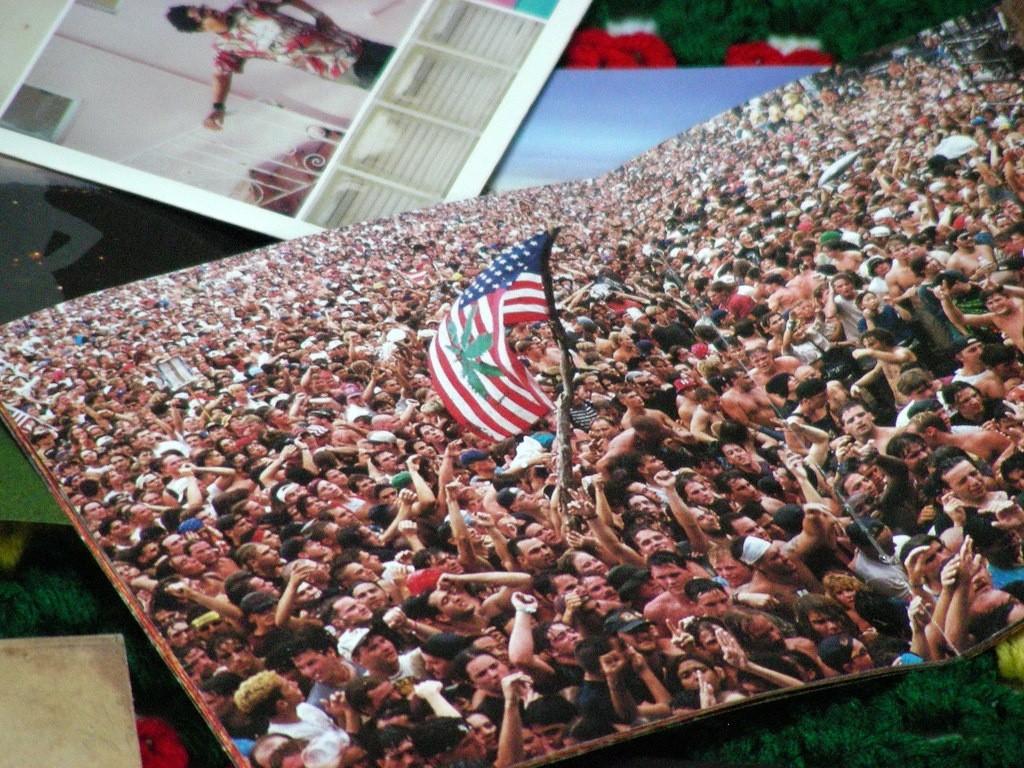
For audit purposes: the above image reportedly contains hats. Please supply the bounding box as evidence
[819,232,840,243]
[336,625,372,662]
[461,448,489,466]
[392,471,412,490]
[603,611,654,639]
[495,487,522,506]
[240,592,277,616]
[907,399,944,419]
[363,431,398,446]
[344,384,362,400]
[674,379,696,394]
[421,633,462,662]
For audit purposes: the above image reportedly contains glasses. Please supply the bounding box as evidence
[199,619,221,633]
[222,645,245,659]
[198,5,208,26]
[269,740,300,768]
[634,379,653,387]
[679,666,706,680]
[955,233,972,243]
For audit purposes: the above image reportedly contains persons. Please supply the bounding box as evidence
[167,0,398,131]
[0,54,1024,768]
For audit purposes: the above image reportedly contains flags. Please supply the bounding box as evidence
[8,409,32,428]
[427,230,558,444]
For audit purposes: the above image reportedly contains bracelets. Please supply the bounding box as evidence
[213,103,225,109]
[515,607,537,614]
[908,581,923,588]
[995,284,1004,293]
[786,320,796,330]
[300,445,308,449]
[505,696,520,706]
[112,412,115,419]
[279,456,287,463]
[583,515,598,520]
